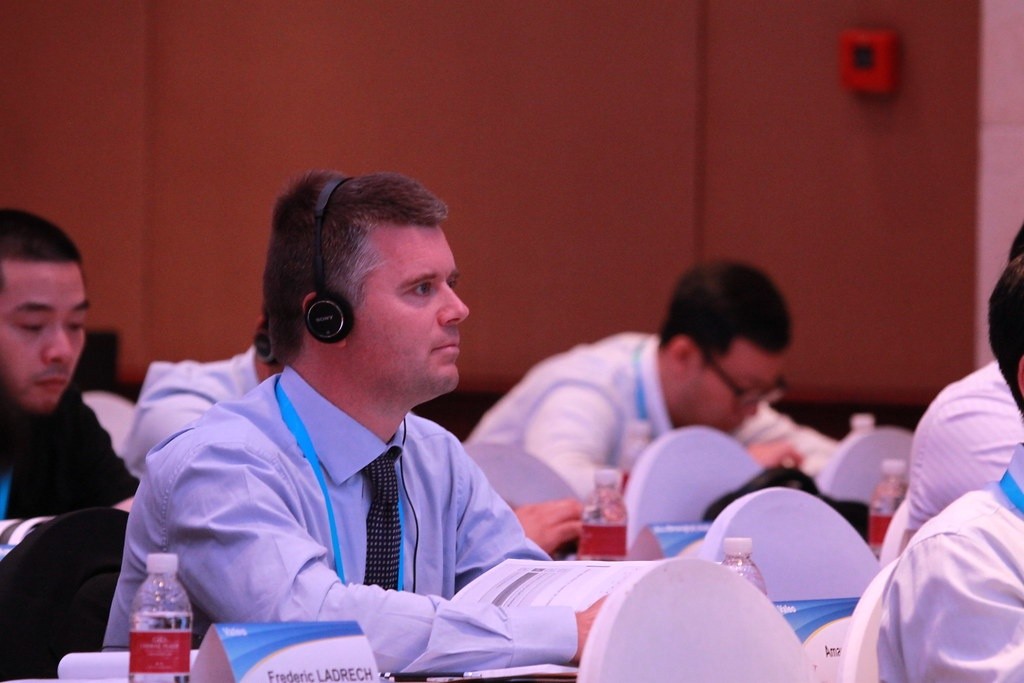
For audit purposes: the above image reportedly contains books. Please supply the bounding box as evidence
[419,559,656,682]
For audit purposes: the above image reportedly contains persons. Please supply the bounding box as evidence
[122,224,1024,683]
[100,166,613,683]
[0,209,138,562]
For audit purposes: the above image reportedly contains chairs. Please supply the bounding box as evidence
[693,487,879,600]
[619,425,763,554]
[576,557,812,683]
[811,428,913,505]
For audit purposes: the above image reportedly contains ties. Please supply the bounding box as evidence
[360,449,401,590]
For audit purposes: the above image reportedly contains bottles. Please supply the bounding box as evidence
[128,552,193,683]
[851,412,876,430]
[716,537,767,597]
[580,468,627,563]
[869,458,910,559]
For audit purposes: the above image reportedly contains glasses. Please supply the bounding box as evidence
[705,348,784,416]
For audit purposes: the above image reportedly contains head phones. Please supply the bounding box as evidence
[306,175,355,344]
